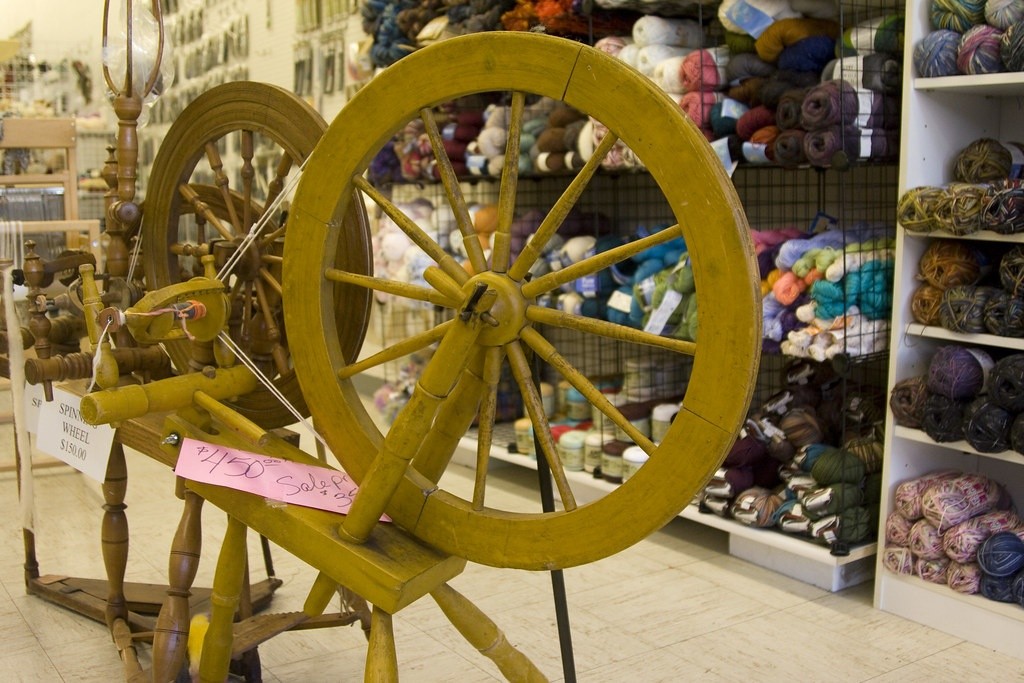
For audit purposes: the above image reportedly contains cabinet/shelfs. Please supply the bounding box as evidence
[359,0,1024,654]
